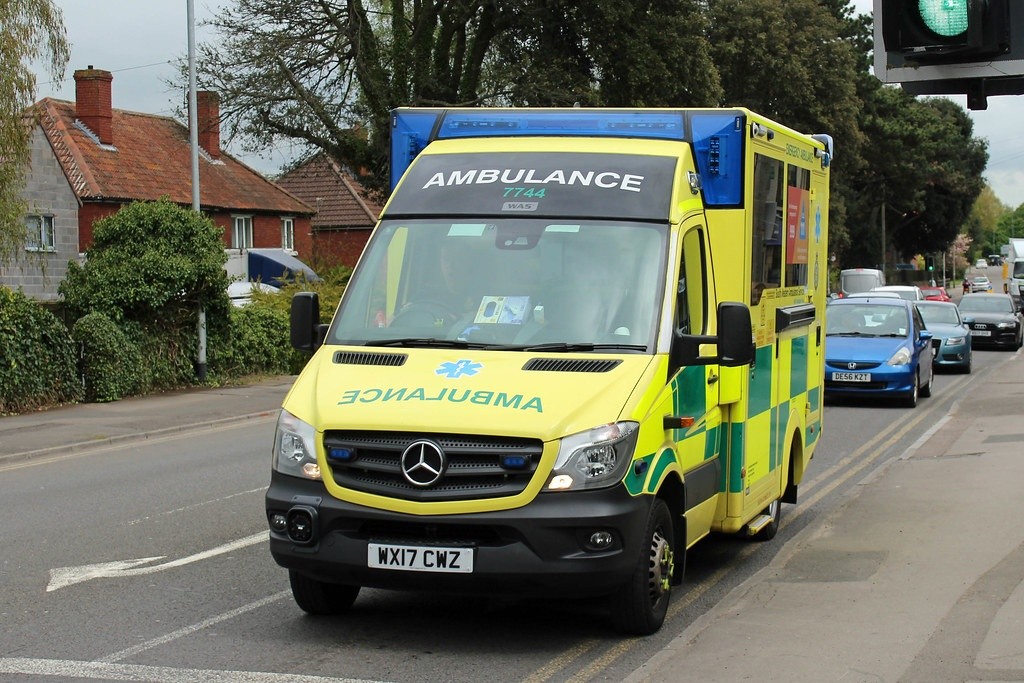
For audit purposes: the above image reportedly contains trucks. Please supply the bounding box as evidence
[221,248,322,288]
[840,269,885,299]
[1003,238,1024,303]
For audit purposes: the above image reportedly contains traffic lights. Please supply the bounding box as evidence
[927,256,934,272]
[882,0,1011,68]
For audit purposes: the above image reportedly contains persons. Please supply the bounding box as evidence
[841,313,853,326]
[962,278,970,288]
[402,246,492,312]
[1002,255,1006,262]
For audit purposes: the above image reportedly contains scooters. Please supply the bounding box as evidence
[962,282,972,295]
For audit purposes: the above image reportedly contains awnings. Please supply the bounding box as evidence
[248,249,320,287]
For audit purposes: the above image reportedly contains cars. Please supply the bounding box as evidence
[989,255,1001,266]
[913,301,976,374]
[845,285,952,305]
[226,283,280,308]
[824,298,932,408]
[975,259,987,270]
[958,293,1024,353]
[971,277,993,293]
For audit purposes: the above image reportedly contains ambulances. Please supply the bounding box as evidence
[264,105,834,638]
